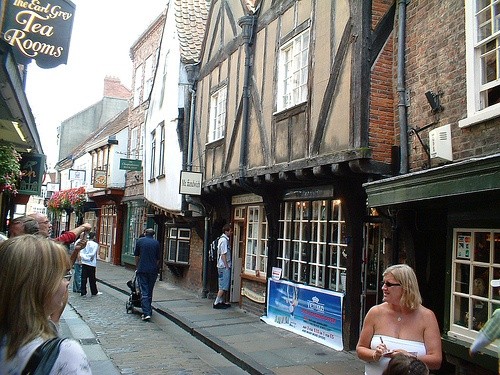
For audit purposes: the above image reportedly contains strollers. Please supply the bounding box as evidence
[125,270,144,316]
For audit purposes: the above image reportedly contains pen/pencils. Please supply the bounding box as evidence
[379,336,384,345]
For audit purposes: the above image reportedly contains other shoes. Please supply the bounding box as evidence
[142,314,151,320]
[213,302,231,309]
[92,292,103,296]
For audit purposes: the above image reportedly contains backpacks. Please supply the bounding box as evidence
[208,237,228,262]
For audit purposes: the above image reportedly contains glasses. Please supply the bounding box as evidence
[381,281,401,287]
[63,270,72,281]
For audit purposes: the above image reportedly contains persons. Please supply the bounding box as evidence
[356,263,442,375]
[383,355,427,375]
[73,232,102,296]
[0,234,91,375]
[134,228,161,320]
[213,226,232,309]
[470,309,500,356]
[0,214,92,323]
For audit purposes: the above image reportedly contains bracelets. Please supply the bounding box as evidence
[373,352,380,361]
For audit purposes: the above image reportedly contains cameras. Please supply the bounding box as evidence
[83,230,89,241]
